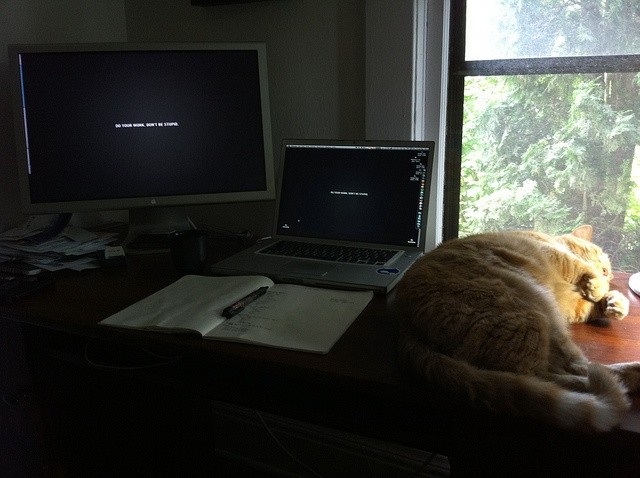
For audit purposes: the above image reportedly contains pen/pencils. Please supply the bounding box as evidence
[222,285,269,319]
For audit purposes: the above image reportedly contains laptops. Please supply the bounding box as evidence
[210,140,435,294]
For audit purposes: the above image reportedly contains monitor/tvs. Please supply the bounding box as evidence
[9,42,277,249]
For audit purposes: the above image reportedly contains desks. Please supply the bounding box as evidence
[0,220,640,478]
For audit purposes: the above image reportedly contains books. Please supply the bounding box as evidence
[97,274,375,355]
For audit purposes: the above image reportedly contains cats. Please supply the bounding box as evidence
[384,225,633,433]
